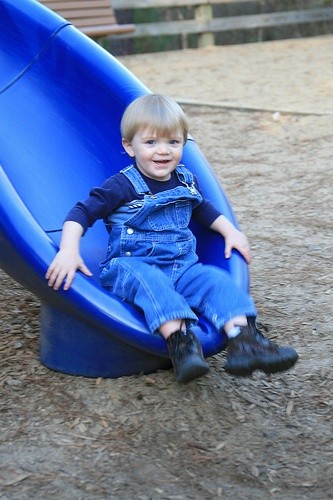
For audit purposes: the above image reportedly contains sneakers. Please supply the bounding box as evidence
[225,322,298,377]
[165,318,210,386]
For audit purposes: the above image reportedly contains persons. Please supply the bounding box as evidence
[45,93,299,384]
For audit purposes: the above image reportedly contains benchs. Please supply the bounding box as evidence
[39,0,135,42]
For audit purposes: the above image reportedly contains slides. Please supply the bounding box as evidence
[0,1,250,377]
